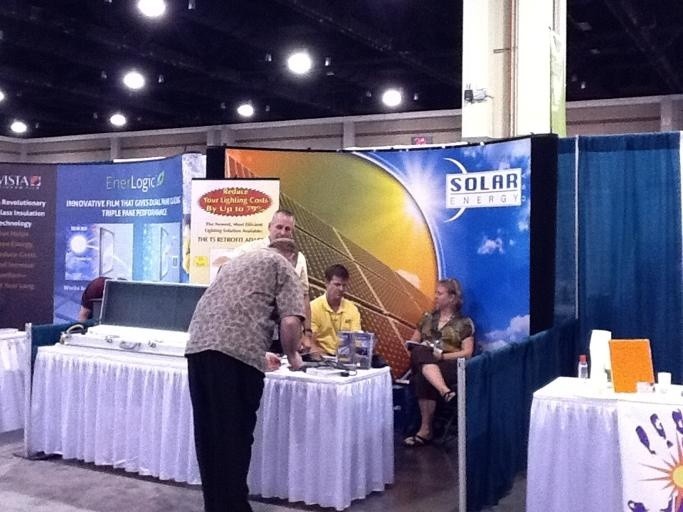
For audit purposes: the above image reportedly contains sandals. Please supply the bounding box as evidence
[404,433,428,446]
[442,390,456,404]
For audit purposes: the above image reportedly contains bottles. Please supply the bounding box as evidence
[578,355,588,384]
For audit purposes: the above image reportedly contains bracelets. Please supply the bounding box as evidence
[440,353,444,361]
[302,328,312,333]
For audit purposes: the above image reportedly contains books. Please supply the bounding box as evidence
[607,338,656,393]
[406,340,434,353]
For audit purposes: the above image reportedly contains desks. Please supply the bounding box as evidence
[524,375,683,512]
[27,332,396,511]
[0,329,26,433]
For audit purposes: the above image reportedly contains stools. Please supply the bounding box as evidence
[439,383,457,447]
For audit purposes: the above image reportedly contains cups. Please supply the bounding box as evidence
[657,372,672,394]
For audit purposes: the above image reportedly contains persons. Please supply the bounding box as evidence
[79,275,127,320]
[184,237,306,512]
[309,264,361,360]
[402,278,475,448]
[212,208,319,350]
